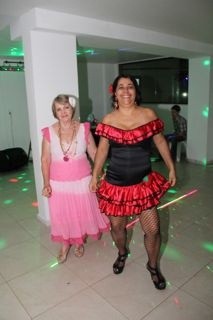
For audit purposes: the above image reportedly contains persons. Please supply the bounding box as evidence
[88,74,177,290]
[40,94,110,263]
[163,104,187,160]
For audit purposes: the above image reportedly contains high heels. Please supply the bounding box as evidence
[146,261,166,290]
[74,243,85,258]
[113,248,129,274]
[56,244,71,264]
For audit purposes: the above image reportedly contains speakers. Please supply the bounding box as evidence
[0,148,28,173]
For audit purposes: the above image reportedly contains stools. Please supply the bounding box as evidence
[167,140,187,163]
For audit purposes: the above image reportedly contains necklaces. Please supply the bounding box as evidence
[57,120,76,160]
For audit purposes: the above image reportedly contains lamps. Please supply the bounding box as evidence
[0,66,24,71]
